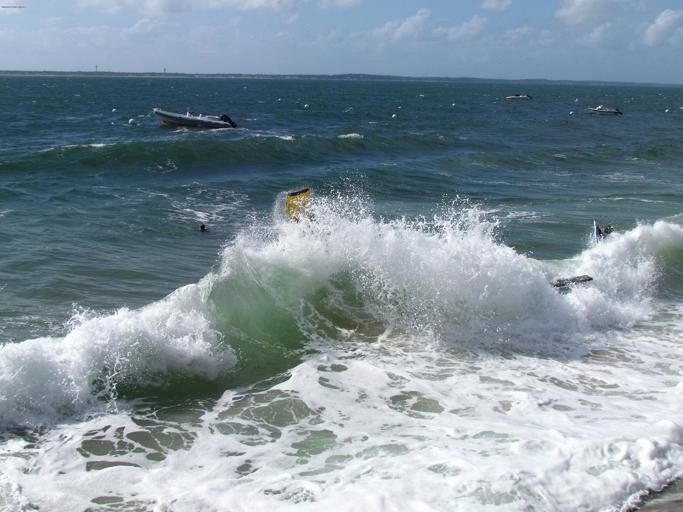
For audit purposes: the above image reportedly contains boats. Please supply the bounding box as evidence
[151,107,236,130]
[505,93,532,100]
[585,106,620,115]
